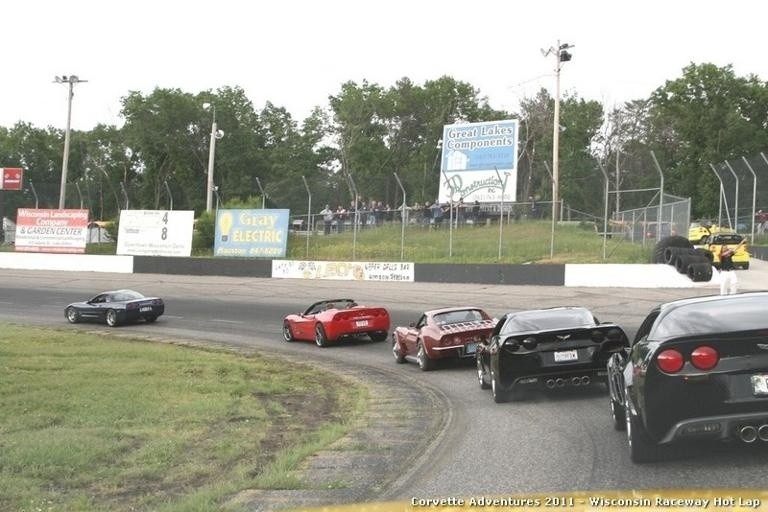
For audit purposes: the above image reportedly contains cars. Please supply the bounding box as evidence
[646,221,678,238]
[688,221,749,270]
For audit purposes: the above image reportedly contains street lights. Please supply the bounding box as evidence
[541,38,574,225]
[202,101,218,213]
[48,74,89,207]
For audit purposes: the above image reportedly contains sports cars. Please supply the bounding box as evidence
[281,297,392,349]
[473,306,632,403]
[390,305,499,372]
[604,289,768,462]
[63,288,165,327]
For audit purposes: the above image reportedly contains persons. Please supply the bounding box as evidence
[755,210,768,235]
[717,236,750,295]
[319,205,333,235]
[335,206,346,232]
[346,196,481,231]
